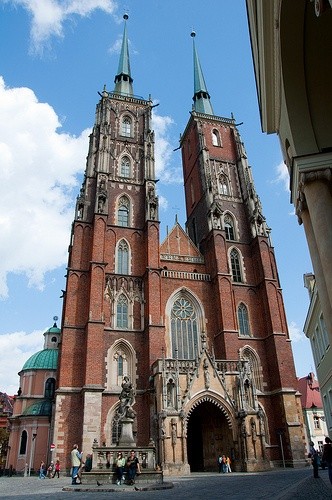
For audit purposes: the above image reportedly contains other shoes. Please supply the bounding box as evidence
[130,481,135,486]
[314,476,320,478]
[118,482,121,485]
[72,483,77,485]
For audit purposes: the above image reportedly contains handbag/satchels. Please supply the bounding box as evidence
[308,453,311,458]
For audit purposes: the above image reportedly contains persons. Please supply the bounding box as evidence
[321,436,332,485]
[308,440,321,478]
[70,443,83,485]
[119,375,136,418]
[126,450,141,485]
[37,460,46,480]
[114,452,125,486]
[78,454,92,478]
[47,461,54,479]
[217,454,233,474]
[51,460,60,478]
